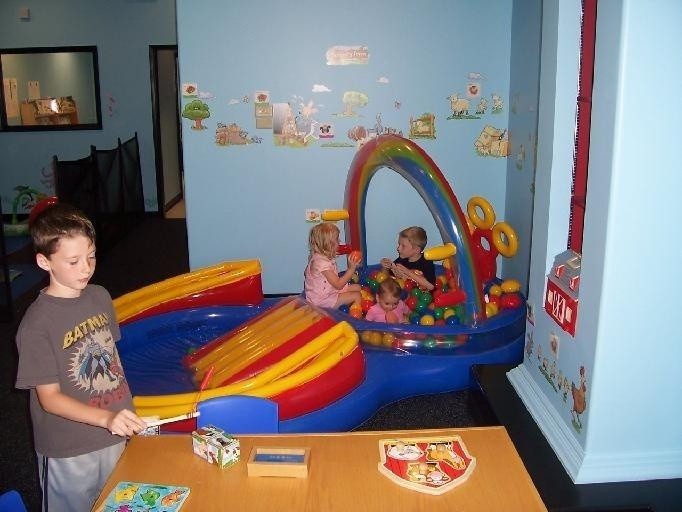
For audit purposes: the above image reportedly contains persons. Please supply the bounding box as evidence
[380,224,439,293]
[12,204,146,511]
[302,220,365,321]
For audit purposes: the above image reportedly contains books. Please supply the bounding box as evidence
[95,478,192,512]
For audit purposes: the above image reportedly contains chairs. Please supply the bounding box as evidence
[196,394,280,433]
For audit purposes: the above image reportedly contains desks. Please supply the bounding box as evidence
[90,425,549,511]
